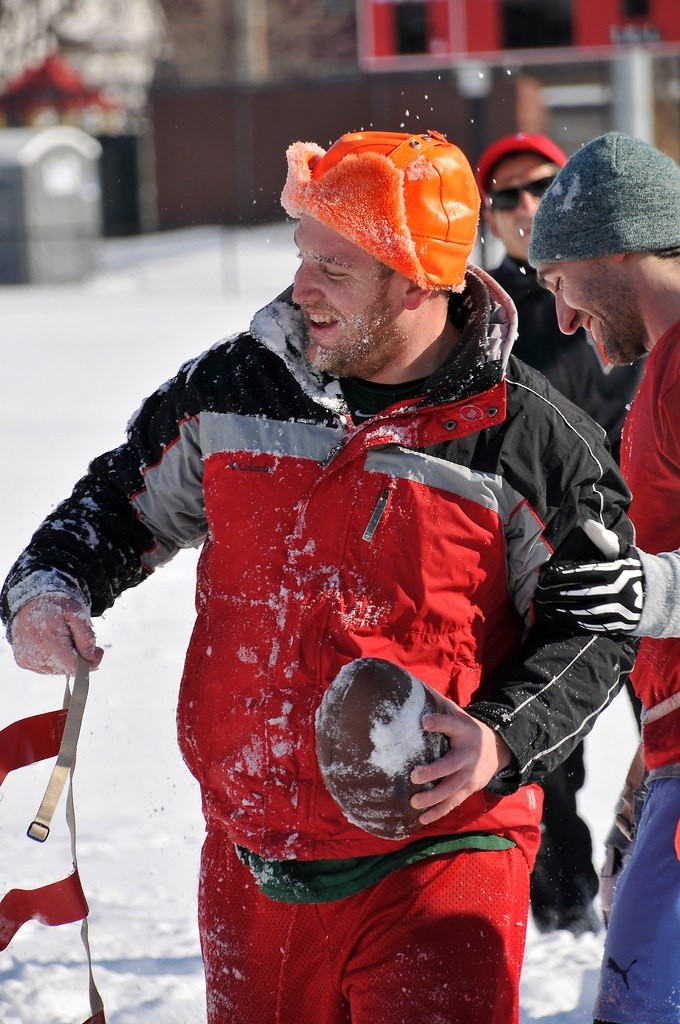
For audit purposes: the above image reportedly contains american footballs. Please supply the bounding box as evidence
[314,657,451,840]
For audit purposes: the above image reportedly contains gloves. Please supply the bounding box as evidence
[534,520,645,632]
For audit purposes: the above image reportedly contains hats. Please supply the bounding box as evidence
[280,131,479,290]
[473,131,568,210]
[526,129,679,266]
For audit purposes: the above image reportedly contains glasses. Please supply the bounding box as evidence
[488,175,560,211]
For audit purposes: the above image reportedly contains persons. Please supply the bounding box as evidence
[530,130,680,1024]
[476,134,650,934]
[0,130,640,1024]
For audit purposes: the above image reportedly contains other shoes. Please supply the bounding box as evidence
[536,901,601,940]
